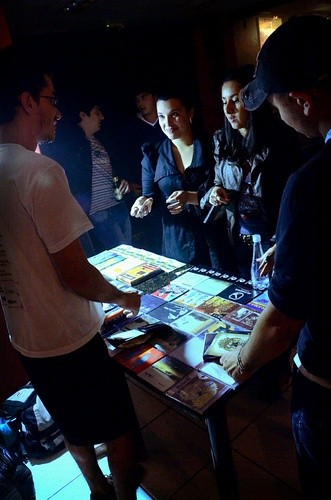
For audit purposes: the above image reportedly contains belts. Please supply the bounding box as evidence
[238,232,271,246]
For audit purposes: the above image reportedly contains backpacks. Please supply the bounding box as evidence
[0,385,65,458]
[0,447,36,500]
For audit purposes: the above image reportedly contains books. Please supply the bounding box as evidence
[87,243,270,415]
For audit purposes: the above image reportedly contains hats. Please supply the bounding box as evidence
[239,15,331,111]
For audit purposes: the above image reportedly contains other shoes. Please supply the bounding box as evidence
[90,474,117,500]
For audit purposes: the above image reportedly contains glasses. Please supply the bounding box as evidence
[38,96,58,106]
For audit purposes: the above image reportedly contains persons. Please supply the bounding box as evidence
[55,99,134,248]
[219,15,331,500]
[209,64,292,278]
[129,87,212,266]
[0,56,140,500]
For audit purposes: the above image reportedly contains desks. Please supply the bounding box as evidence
[87,244,293,500]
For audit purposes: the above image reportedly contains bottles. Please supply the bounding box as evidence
[113,175,125,202]
[250,234,270,290]
